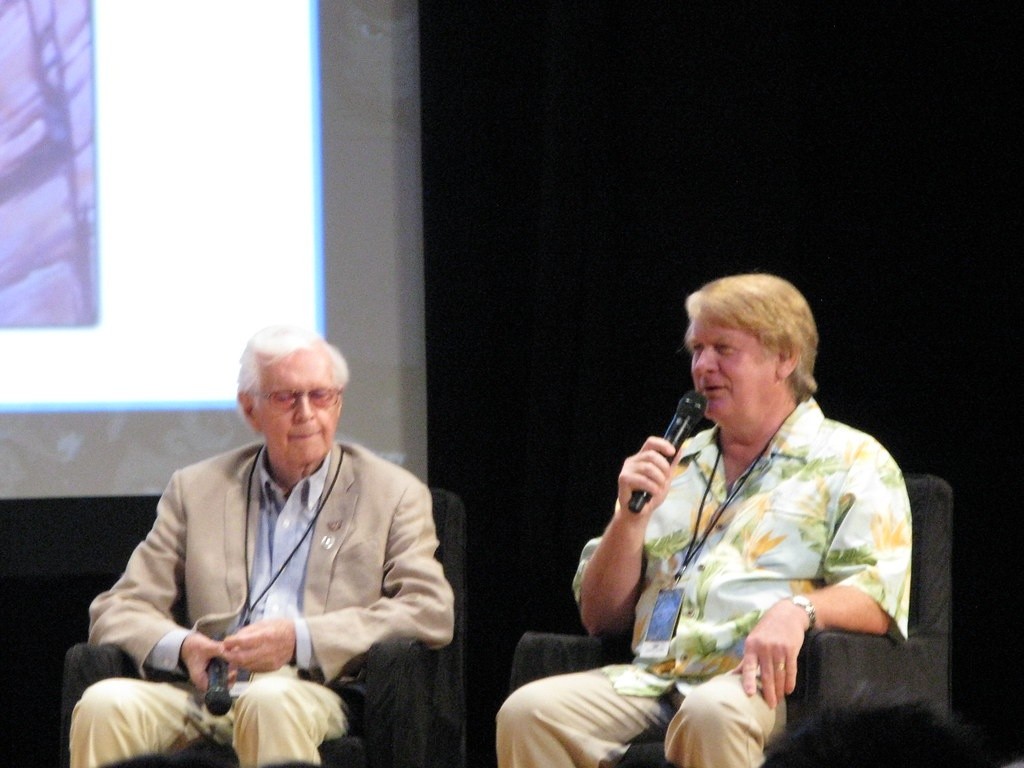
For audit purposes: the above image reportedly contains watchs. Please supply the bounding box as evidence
[783,595,816,630]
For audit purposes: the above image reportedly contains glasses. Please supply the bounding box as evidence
[260,386,343,415]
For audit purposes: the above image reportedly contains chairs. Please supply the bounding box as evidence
[513,471,952,768]
[59,489,468,768]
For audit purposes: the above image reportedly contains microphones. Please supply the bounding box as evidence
[628,389,709,514]
[203,657,232,718]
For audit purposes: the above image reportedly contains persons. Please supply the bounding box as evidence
[496,273,912,768]
[70,325,454,768]
